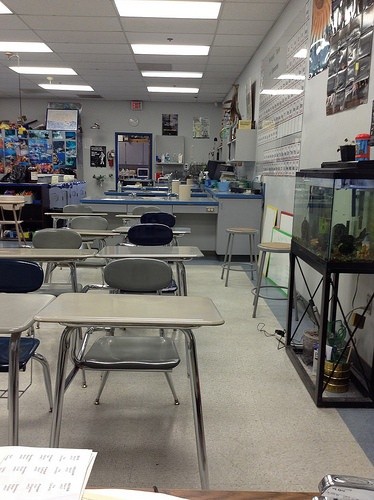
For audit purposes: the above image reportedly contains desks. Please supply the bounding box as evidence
[112,227,192,246]
[115,214,177,226]
[36,229,120,248]
[96,245,204,336]
[0,248,97,337]
[0,200,28,245]
[0,293,56,446]
[34,292,224,491]
[43,212,108,230]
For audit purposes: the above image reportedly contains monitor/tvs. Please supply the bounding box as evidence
[46,108,79,130]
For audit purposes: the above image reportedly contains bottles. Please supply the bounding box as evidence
[313,345,318,377]
[117,181,121,193]
[160,153,183,164]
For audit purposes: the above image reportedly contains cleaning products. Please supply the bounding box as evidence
[203,171,211,188]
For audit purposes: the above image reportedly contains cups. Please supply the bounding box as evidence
[217,182,230,191]
[178,185,192,201]
[186,179,193,185]
[172,180,181,194]
[51,175,59,186]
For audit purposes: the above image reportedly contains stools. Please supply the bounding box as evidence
[253,242,299,321]
[220,227,259,288]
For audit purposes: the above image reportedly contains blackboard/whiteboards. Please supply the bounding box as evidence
[256,205,294,297]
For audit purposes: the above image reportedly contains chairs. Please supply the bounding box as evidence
[0,194,225,491]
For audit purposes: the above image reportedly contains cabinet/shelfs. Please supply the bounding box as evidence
[208,119,257,163]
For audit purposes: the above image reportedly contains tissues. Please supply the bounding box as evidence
[216,177,231,192]
